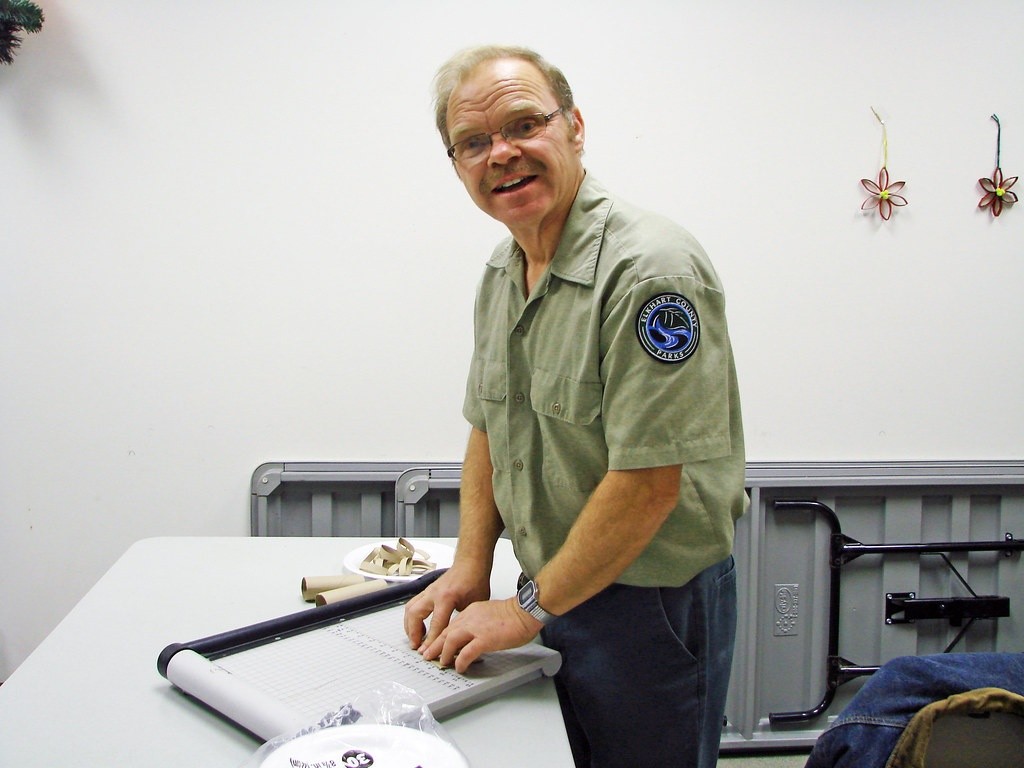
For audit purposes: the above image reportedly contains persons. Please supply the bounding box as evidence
[404,46,745,768]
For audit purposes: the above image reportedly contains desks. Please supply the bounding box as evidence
[0,534,576,768]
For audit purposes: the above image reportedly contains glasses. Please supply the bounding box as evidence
[447,106,564,169]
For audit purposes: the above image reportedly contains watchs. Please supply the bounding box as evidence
[518,576,558,625]
